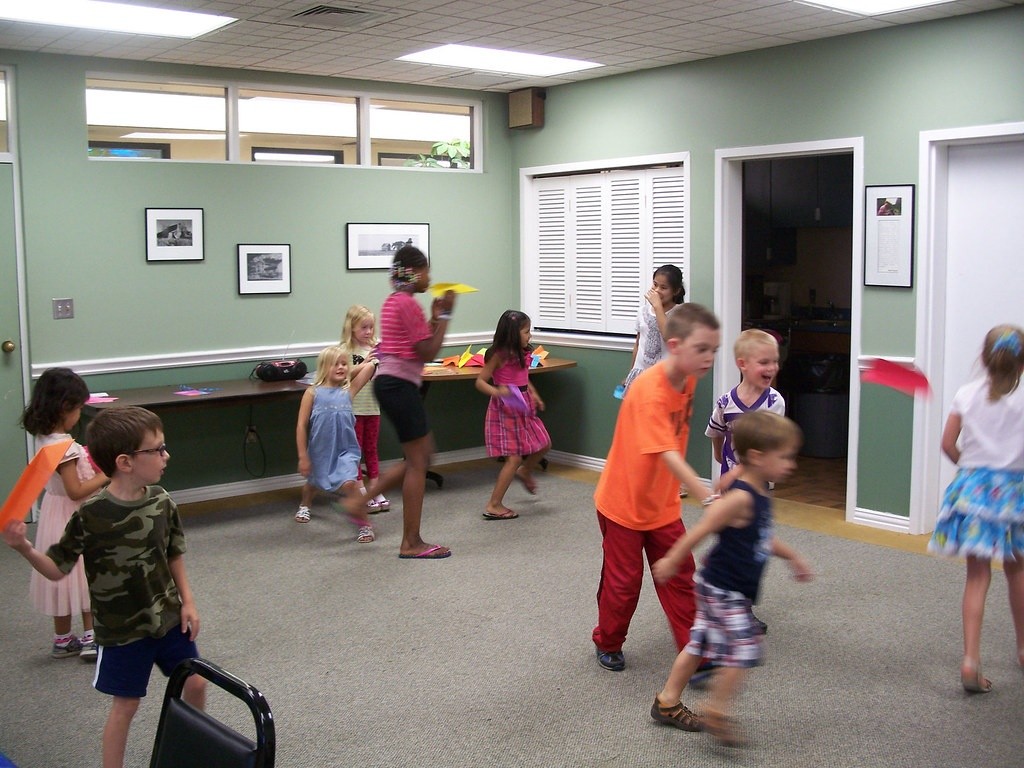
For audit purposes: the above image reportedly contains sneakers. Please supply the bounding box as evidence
[689,665,718,686]
[79,636,98,660]
[595,647,626,671]
[51,636,81,658]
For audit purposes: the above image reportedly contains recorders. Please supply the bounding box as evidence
[256,330,307,381]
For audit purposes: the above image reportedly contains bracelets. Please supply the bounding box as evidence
[701,494,720,505]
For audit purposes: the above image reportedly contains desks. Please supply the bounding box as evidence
[73,356,579,507]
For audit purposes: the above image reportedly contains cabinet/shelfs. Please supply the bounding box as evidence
[743,154,851,232]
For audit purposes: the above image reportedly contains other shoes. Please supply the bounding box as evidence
[378,500,389,512]
[367,502,382,514]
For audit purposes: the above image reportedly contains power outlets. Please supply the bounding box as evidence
[244,425,257,444]
[808,289,817,304]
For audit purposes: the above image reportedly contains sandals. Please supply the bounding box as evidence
[962,664,993,693]
[357,523,375,543]
[650,691,705,732]
[294,504,311,523]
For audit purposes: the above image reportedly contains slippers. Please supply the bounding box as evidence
[399,545,451,559]
[515,472,537,495]
[482,508,518,520]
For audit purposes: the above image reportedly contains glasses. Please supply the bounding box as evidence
[133,443,166,458]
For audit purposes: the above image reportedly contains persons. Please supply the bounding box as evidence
[22,368,112,661]
[476,310,552,519]
[876,201,900,215]
[651,411,813,747]
[705,329,786,507]
[294,346,375,544]
[942,324,1024,693]
[621,264,688,499]
[4,405,208,768]
[592,303,719,689]
[340,248,455,558]
[337,305,391,514]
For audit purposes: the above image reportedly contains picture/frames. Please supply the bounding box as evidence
[863,183,915,289]
[237,244,292,295]
[145,207,205,262]
[346,222,430,271]
